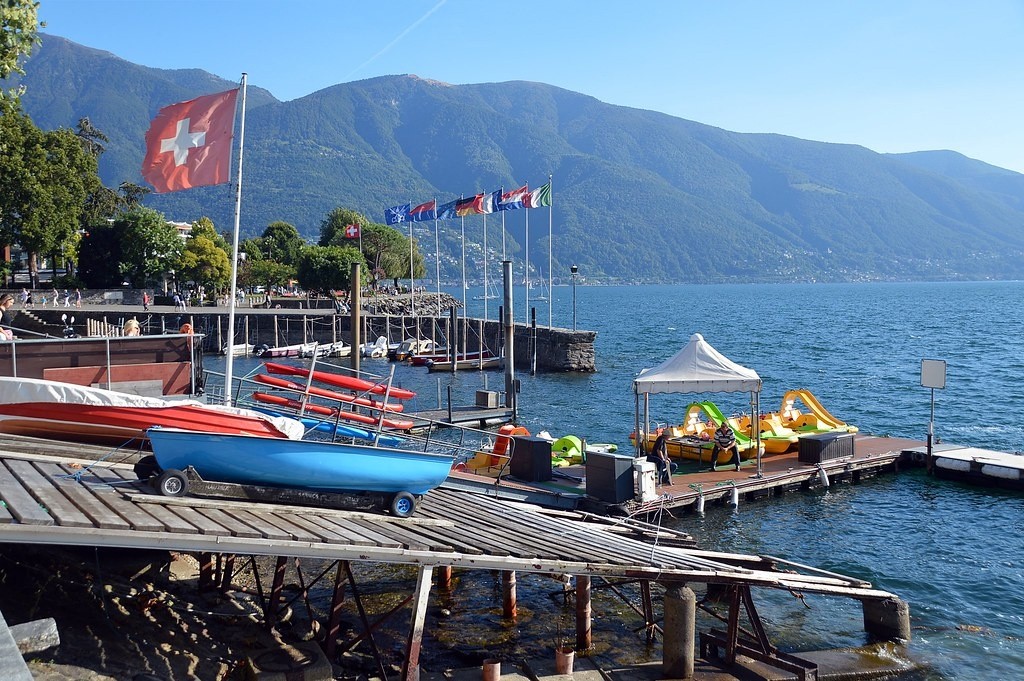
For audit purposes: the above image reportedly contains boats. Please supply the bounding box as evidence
[222,342,255,356]
[411,350,492,366]
[327,339,452,362]
[451,421,620,497]
[257,340,321,359]
[0,374,304,445]
[425,355,507,372]
[725,386,858,454]
[627,399,766,462]
[296,340,343,358]
[324,342,375,358]
[145,421,458,497]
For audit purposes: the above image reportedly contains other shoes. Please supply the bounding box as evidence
[735,466,740,472]
[662,479,675,485]
[710,464,716,471]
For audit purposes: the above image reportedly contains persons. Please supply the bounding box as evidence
[41,295,47,308]
[64,290,71,307]
[0,293,14,340]
[124,320,145,337]
[74,288,82,308]
[240,289,244,303]
[710,421,741,471]
[405,286,423,294]
[51,287,59,307]
[179,324,194,351]
[173,291,203,313]
[18,288,35,309]
[142,292,149,310]
[651,429,678,482]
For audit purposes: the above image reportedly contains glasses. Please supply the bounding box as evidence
[721,425,727,428]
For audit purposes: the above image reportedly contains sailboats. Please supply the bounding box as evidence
[472,271,501,300]
[528,267,550,301]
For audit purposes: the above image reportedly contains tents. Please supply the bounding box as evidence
[635,334,765,478]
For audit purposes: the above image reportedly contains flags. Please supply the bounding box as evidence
[500,185,528,210]
[482,189,502,215]
[437,197,463,220]
[141,88,239,192]
[383,204,410,226]
[522,181,551,209]
[456,193,484,217]
[345,224,359,239]
[408,200,435,222]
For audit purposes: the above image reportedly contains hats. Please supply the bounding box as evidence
[662,430,672,436]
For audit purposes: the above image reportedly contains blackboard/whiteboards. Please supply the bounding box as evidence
[920,359,946,389]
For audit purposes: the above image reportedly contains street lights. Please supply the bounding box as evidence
[570,265,578,332]
